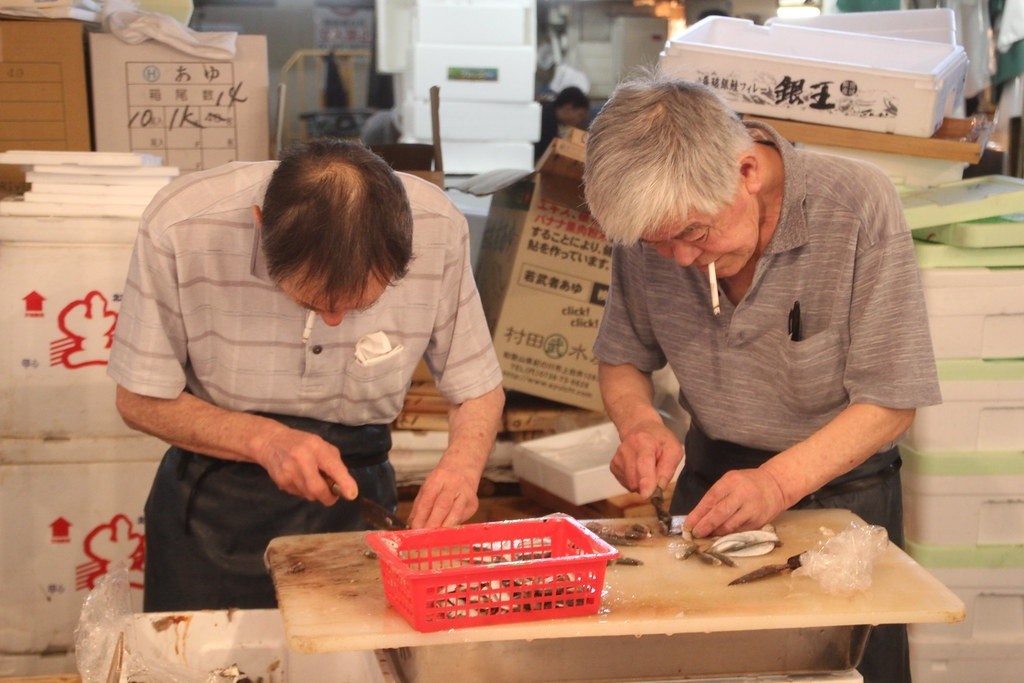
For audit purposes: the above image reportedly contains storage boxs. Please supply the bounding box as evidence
[1,19,270,654]
[457,11,993,413]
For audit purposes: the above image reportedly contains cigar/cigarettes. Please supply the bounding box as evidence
[302,308,316,343]
[708,260,721,316]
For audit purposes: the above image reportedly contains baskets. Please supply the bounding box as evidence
[364,514,621,634]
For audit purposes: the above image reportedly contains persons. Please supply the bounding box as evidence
[582,62,943,683]
[107,137,507,614]
[534,85,589,167]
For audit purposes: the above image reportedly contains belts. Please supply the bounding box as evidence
[806,457,902,503]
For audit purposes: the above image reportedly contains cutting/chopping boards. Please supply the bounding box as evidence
[267,508,967,654]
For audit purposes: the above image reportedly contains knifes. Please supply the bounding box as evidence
[728,550,805,586]
[322,474,411,531]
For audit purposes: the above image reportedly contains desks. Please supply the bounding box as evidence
[269,508,968,682]
